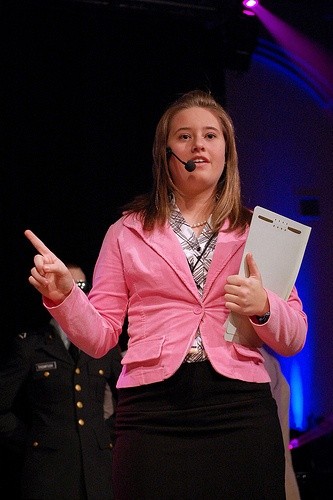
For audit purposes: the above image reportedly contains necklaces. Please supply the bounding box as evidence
[191,221,207,228]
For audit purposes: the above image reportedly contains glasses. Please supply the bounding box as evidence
[76,281,85,290]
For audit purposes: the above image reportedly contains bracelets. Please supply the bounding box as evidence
[255,312,270,324]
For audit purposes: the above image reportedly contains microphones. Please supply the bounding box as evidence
[165,147,196,172]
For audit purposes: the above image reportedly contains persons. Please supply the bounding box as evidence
[24,89,308,500]
[0,261,129,500]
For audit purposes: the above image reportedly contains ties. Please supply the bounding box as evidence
[68,341,80,365]
